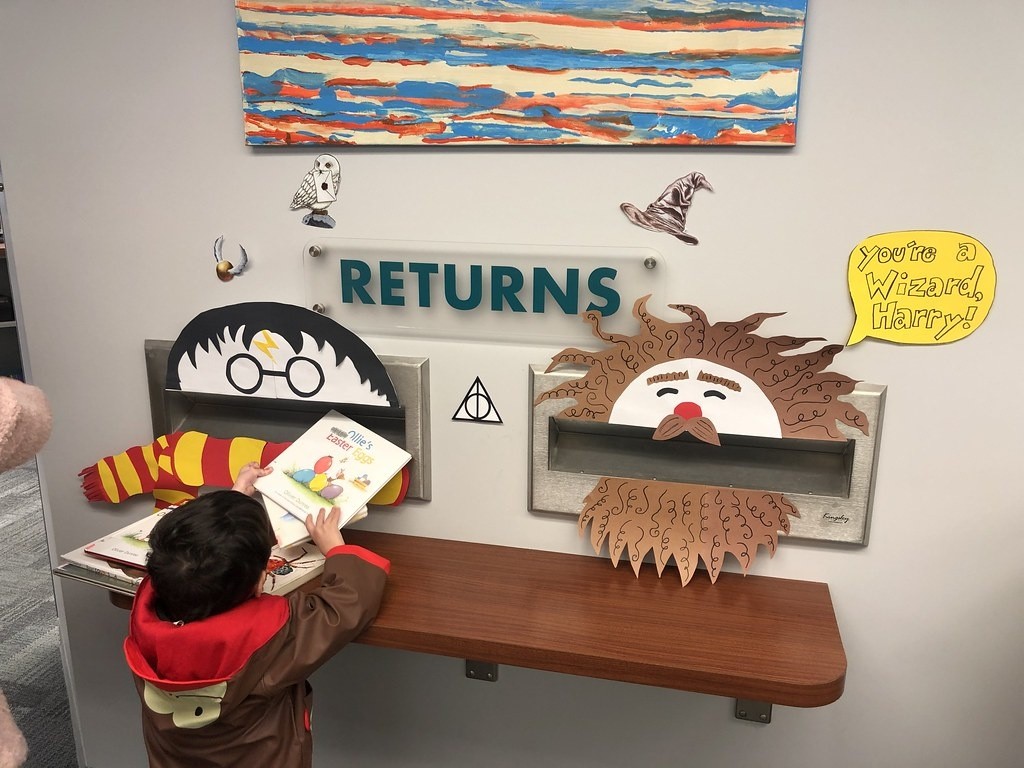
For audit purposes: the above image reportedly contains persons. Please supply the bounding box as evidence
[124,462,391,768]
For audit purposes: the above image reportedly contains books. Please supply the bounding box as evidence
[52,503,327,601]
[258,491,369,551]
[252,409,413,531]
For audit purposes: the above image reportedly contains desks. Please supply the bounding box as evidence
[109,528,847,724]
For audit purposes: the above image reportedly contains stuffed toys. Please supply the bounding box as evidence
[0,378,52,768]
